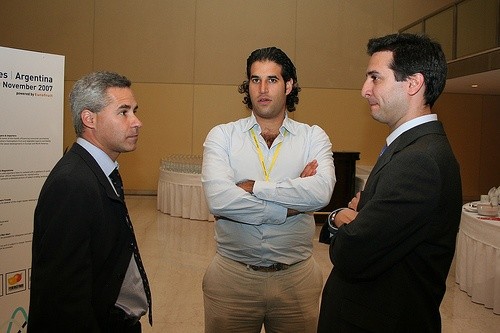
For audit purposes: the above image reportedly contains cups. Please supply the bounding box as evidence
[480,195,490,203]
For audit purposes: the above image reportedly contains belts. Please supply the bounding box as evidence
[233,259,302,272]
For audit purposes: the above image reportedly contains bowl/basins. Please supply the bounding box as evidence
[477,203,500,216]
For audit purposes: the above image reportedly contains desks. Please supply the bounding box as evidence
[317,152,361,214]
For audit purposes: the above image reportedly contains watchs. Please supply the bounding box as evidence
[331,209,342,226]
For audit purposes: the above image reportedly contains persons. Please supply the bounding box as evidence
[316,34,463,333]
[26,70,154,333]
[201,47,337,333]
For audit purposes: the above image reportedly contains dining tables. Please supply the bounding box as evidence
[454,200,499,315]
[156,155,216,222]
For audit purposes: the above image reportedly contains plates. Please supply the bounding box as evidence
[466,203,478,212]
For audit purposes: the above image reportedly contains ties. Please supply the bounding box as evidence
[108,168,153,327]
[377,141,388,160]
